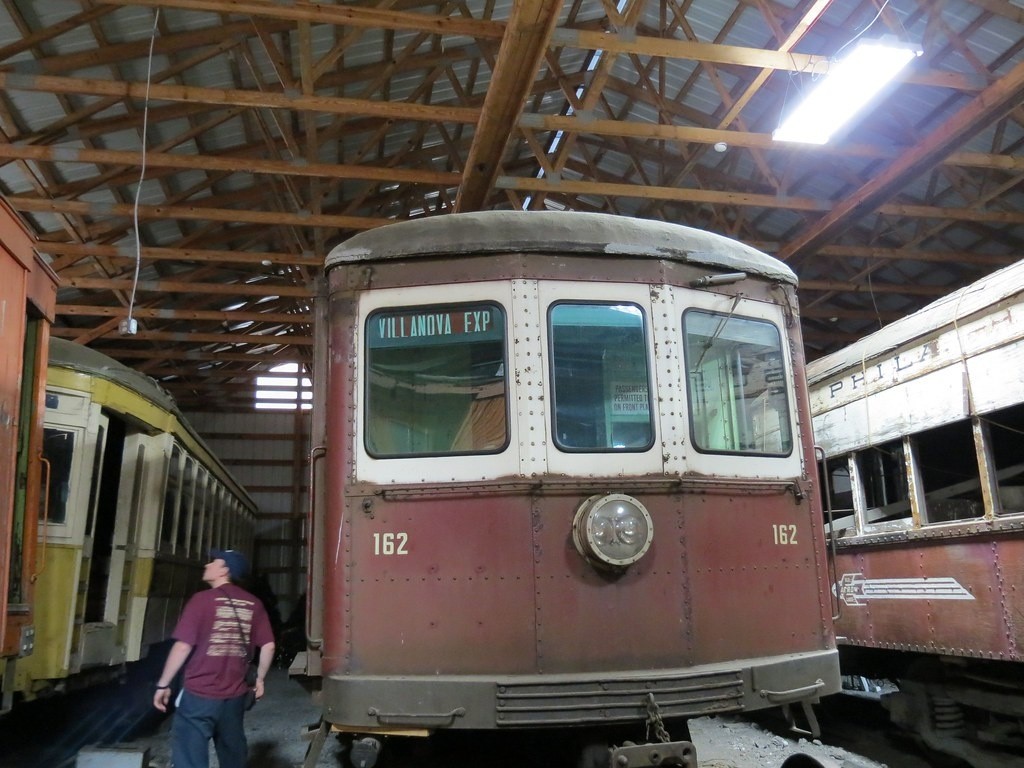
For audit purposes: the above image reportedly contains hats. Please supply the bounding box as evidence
[212,550,243,584]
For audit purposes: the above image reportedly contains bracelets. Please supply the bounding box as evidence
[156,685,170,689]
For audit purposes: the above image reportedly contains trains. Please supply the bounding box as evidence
[291,218,846,766]
[1,194,261,706]
[806,261,1024,768]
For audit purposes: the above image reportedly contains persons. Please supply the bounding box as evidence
[152,550,275,768]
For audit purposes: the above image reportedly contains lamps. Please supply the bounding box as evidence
[769,37,924,147]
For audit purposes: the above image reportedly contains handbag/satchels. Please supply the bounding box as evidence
[245,663,257,686]
